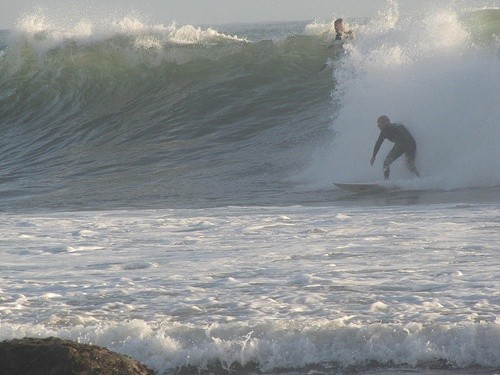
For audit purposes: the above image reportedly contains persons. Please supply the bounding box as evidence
[369,114,421,187]
[317,17,356,72]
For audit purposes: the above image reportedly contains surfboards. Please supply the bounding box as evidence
[334,177,425,191]
